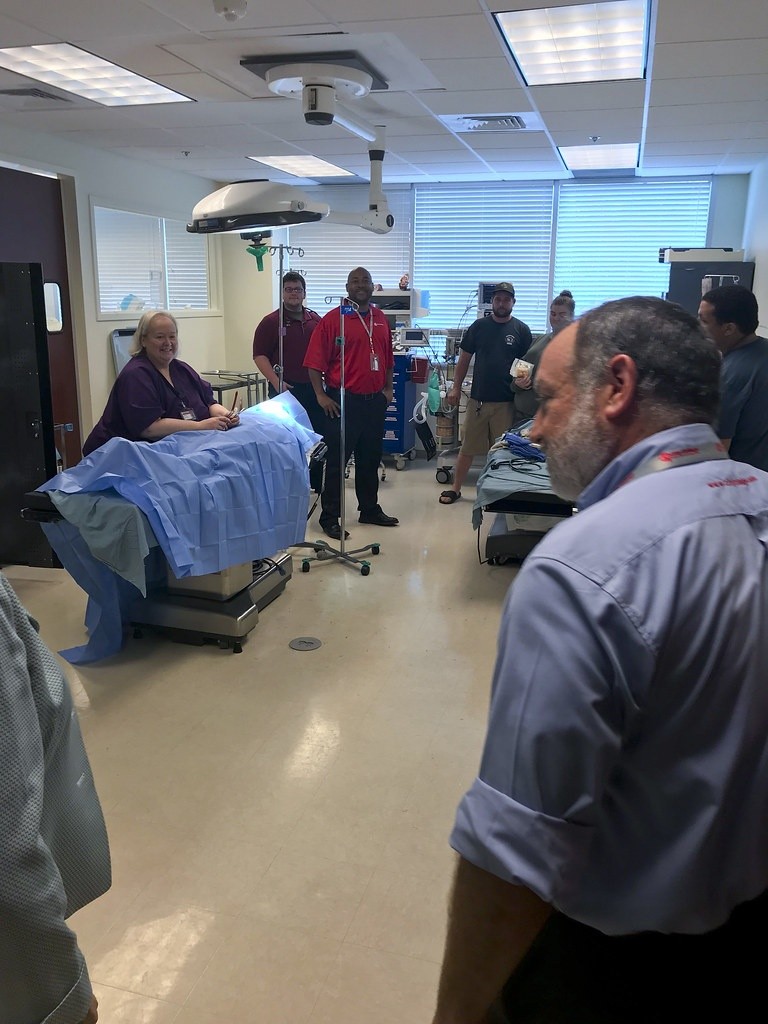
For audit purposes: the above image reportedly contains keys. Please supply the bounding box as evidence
[475,408,480,416]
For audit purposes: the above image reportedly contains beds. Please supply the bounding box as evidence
[22,389,328,656]
[470,417,579,566]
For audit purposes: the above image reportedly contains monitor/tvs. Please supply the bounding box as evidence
[479,281,498,310]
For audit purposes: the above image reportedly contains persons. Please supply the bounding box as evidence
[303,267,400,540]
[436,296,768,1024]
[253,273,326,492]
[439,282,532,504]
[83,311,240,458]
[0,571,113,1024]
[695,285,768,472]
[510,291,575,425]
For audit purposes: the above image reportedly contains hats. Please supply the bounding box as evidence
[493,282,515,295]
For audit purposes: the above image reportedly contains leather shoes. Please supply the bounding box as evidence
[358,504,399,526]
[320,524,350,540]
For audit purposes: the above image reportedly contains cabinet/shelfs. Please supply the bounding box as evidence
[382,351,417,470]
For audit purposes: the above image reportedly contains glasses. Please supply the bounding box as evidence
[284,287,305,293]
[493,297,512,304]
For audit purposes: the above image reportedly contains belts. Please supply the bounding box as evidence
[326,387,382,401]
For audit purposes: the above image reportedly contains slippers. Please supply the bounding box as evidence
[439,491,461,504]
